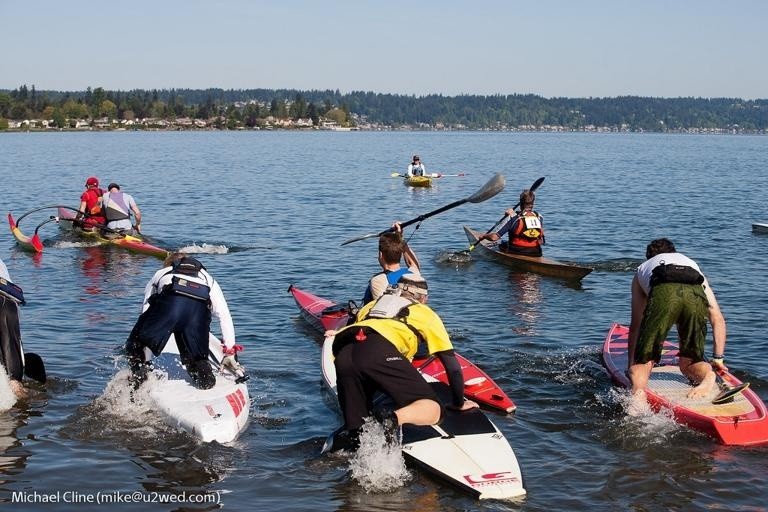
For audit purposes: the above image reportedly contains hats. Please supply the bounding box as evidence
[85,177,99,186]
[397,273,428,295]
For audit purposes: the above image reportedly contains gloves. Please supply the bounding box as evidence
[219,356,246,379]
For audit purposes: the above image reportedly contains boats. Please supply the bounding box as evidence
[463,224,594,280]
[140,331,251,446]
[287,284,518,415]
[57,205,173,260]
[405,175,433,188]
[601,321,768,447]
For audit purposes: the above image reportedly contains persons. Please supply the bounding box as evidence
[0,291,48,398]
[407,154,426,178]
[122,250,245,395]
[90,181,141,238]
[317,285,467,460]
[362,221,428,305]
[72,177,107,234]
[475,190,544,258]
[624,236,729,401]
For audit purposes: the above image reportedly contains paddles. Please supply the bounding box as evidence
[450,177,545,262]
[703,352,749,403]
[339,173,505,245]
[55,215,141,242]
[391,172,464,178]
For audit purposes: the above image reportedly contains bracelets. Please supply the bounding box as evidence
[712,353,726,359]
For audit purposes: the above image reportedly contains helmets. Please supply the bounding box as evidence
[413,156,419,161]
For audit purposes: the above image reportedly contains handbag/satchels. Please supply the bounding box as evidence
[171,276,210,300]
[649,263,704,287]
[0,276,24,303]
